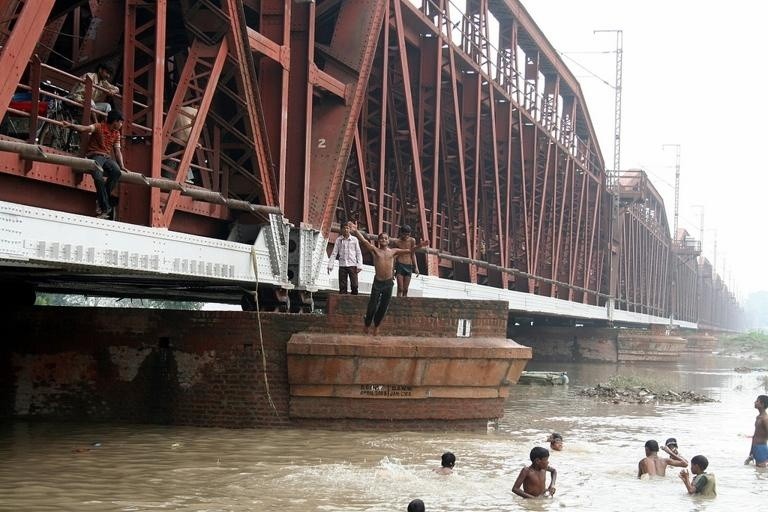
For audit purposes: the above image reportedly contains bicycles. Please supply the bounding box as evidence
[37,80,82,155]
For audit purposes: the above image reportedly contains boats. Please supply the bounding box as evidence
[518,371,570,386]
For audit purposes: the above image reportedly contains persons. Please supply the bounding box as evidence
[547,433,562,451]
[408,499,425,512]
[327,220,430,336]
[638,438,715,496]
[172,105,203,184]
[62,57,131,219]
[745,395,768,467]
[433,452,456,474]
[512,448,557,498]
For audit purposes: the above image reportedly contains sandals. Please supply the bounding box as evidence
[96,200,112,218]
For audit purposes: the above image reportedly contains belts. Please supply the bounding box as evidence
[95,154,104,157]
